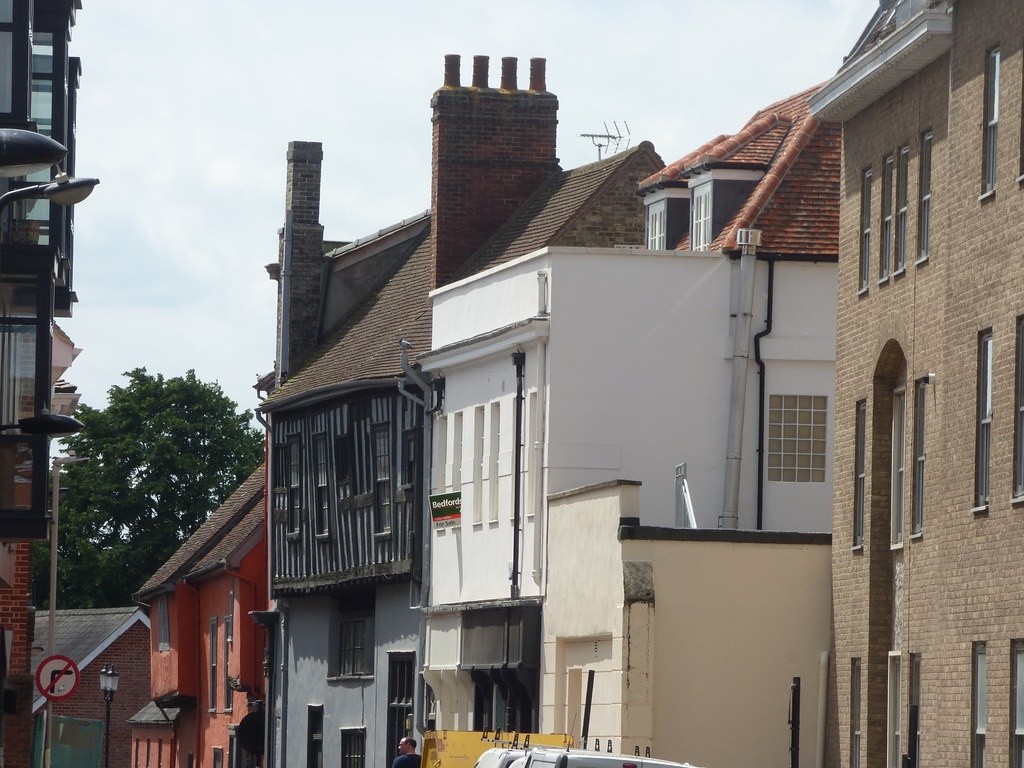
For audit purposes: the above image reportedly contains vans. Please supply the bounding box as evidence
[474,748,694,768]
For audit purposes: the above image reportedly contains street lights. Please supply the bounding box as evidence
[99,661,119,768]
[41,456,93,765]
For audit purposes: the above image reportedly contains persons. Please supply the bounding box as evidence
[392,737,422,768]
